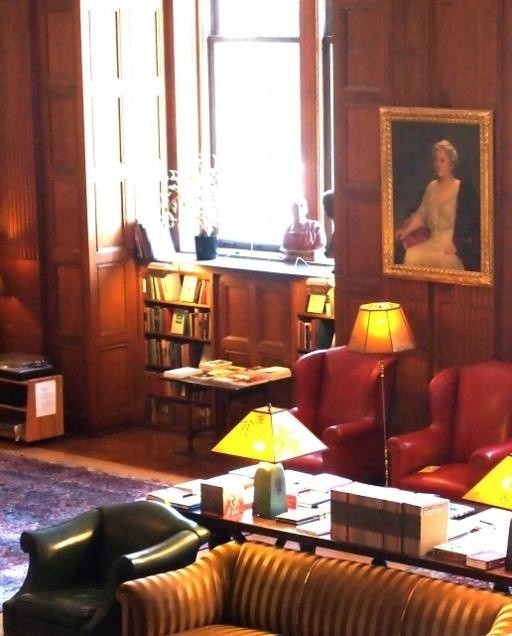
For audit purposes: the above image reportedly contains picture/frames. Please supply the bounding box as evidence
[377,101,497,290]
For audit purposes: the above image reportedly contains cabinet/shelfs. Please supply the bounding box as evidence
[0,372,64,448]
[203,266,301,371]
[291,275,340,367]
[141,251,217,439]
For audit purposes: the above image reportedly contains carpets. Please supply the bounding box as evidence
[1,451,254,605]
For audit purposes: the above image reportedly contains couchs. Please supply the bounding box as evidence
[0,497,213,634]
[233,340,397,480]
[385,360,512,512]
[107,537,512,635]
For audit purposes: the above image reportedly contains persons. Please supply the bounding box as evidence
[394,138,479,272]
[320,189,338,260]
[282,197,325,251]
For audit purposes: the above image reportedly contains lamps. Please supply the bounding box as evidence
[212,402,330,519]
[461,452,512,575]
[346,297,416,485]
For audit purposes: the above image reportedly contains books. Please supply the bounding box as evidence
[143,271,337,431]
[133,220,178,262]
[145,457,512,570]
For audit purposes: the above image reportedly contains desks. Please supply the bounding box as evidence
[136,461,512,592]
[158,366,284,457]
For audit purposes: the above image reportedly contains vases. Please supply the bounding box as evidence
[193,235,219,260]
